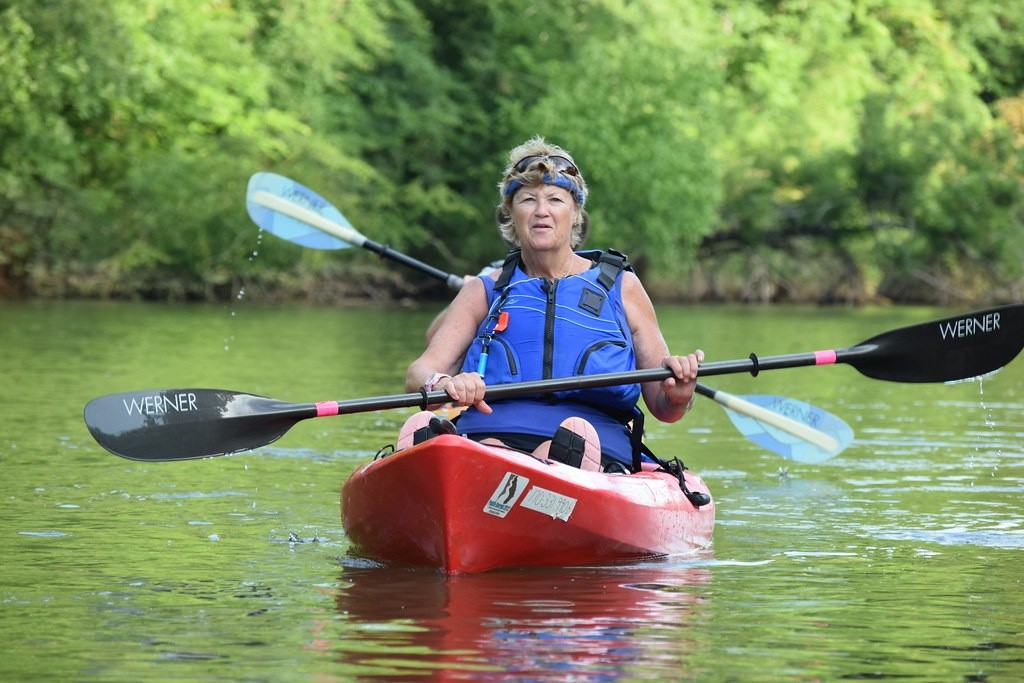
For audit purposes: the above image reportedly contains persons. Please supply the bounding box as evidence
[398,136,705,476]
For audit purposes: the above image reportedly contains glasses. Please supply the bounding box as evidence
[513,155,578,173]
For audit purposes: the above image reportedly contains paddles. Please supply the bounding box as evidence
[245,172,855,464]
[82,301,1024,463]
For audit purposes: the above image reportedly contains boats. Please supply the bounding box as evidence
[340,434,715,576]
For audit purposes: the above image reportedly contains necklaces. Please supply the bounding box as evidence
[566,253,573,276]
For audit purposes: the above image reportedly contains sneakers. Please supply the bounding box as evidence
[396,411,440,452]
[548,417,601,473]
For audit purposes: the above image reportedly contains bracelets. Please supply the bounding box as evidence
[663,393,695,413]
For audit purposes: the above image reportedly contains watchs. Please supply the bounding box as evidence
[424,373,452,406]
